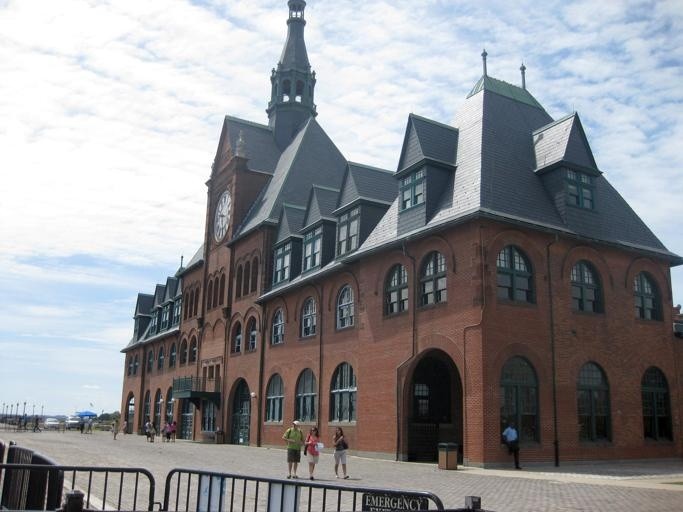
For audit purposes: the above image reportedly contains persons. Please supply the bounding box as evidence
[170,421,176,442]
[150,421,155,442]
[332,426,349,479]
[165,422,172,442]
[304,426,321,480]
[113,417,120,440]
[23,413,28,431]
[502,418,524,472]
[159,421,166,442]
[145,419,151,442]
[78,417,83,432]
[121,419,126,433]
[84,417,92,434]
[33,414,41,432]
[281,421,305,479]
[15,414,21,432]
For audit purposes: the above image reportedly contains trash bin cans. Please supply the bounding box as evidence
[438,443,458,470]
[215,431,224,444]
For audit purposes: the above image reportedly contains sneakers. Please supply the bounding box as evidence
[286,473,350,480]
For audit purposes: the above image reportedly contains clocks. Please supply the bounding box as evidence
[212,189,231,243]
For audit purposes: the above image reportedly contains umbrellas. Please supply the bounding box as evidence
[72,409,96,417]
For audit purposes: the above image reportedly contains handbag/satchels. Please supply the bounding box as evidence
[341,440,348,449]
[303,434,310,456]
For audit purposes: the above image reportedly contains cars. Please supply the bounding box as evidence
[43,418,60,429]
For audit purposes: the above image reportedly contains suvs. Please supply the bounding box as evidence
[65,416,81,430]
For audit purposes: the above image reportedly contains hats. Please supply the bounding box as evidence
[292,420,300,426]
